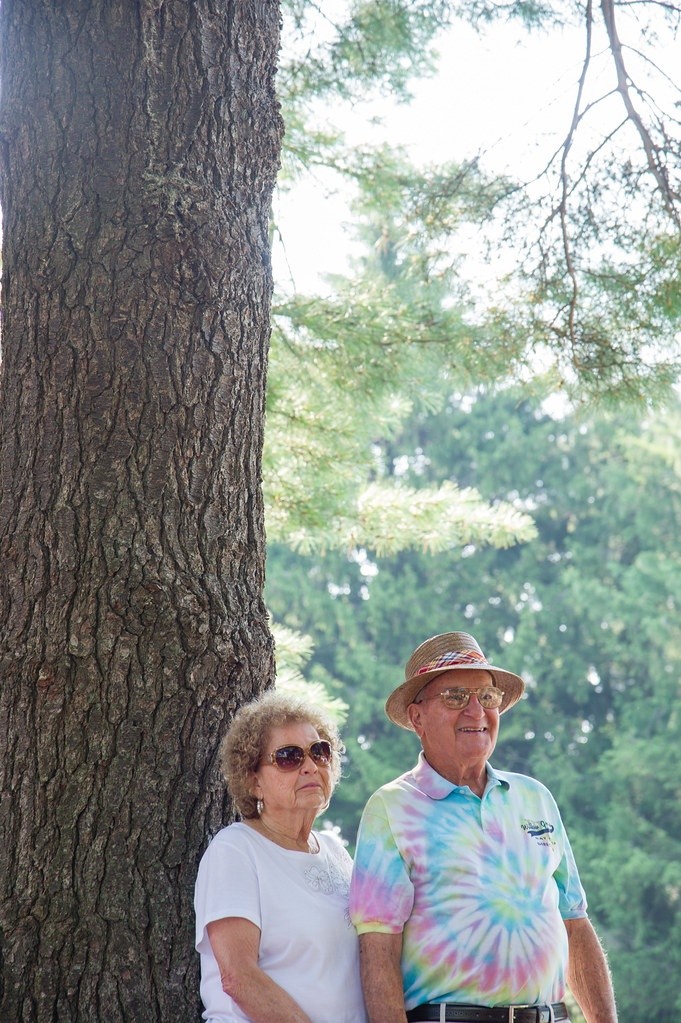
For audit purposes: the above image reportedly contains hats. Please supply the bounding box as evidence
[386,632,524,731]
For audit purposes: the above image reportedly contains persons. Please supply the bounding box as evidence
[192,690,372,1023]
[348,630,622,1023]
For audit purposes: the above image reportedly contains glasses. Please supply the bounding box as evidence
[418,686,505,710]
[256,740,332,772]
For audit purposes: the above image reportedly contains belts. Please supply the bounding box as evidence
[406,1002,567,1023]
